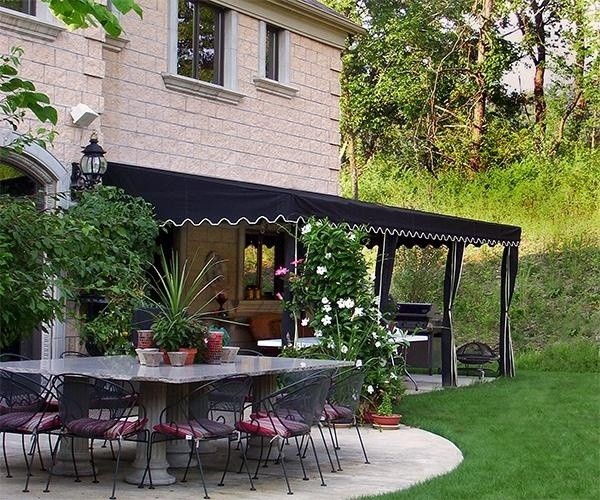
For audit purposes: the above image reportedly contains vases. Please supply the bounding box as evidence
[137,330,154,349]
[135,349,159,364]
[205,332,224,365]
[142,353,164,367]
[167,352,188,367]
[220,347,240,363]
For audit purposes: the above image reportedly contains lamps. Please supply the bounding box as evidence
[70,129,107,202]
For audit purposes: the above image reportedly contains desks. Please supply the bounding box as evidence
[257,335,428,391]
[0,355,355,486]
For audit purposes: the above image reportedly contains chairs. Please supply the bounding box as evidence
[28,351,134,455]
[237,349,371,474]
[207,349,263,450]
[0,354,82,492]
[43,372,155,499]
[217,354,327,495]
[138,373,256,499]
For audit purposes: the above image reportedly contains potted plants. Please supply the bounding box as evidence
[363,412,377,422]
[303,312,378,428]
[139,246,249,365]
[372,391,402,430]
